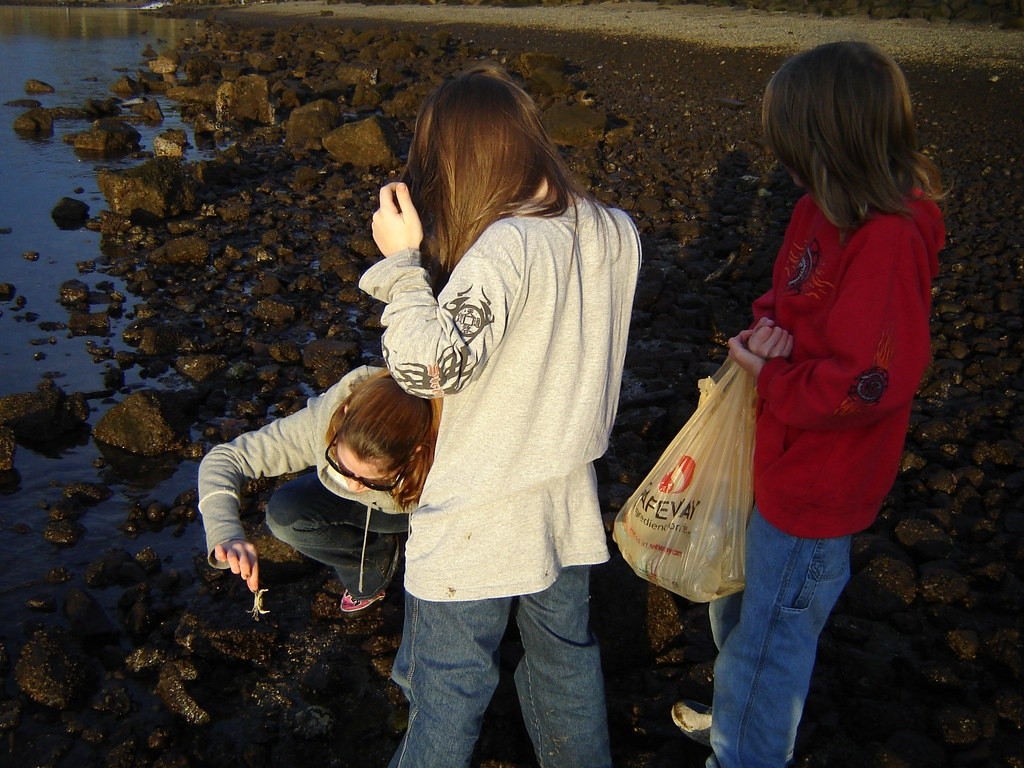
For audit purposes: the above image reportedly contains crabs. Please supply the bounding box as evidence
[246,588,271,620]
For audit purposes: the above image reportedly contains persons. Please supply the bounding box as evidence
[197,364,439,613]
[667,40,942,765]
[357,67,644,765]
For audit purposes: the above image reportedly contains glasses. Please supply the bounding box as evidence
[326,430,409,493]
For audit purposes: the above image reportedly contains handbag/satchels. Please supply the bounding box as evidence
[614,351,756,604]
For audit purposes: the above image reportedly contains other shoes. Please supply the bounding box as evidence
[671,698,714,748]
[340,586,386,613]
